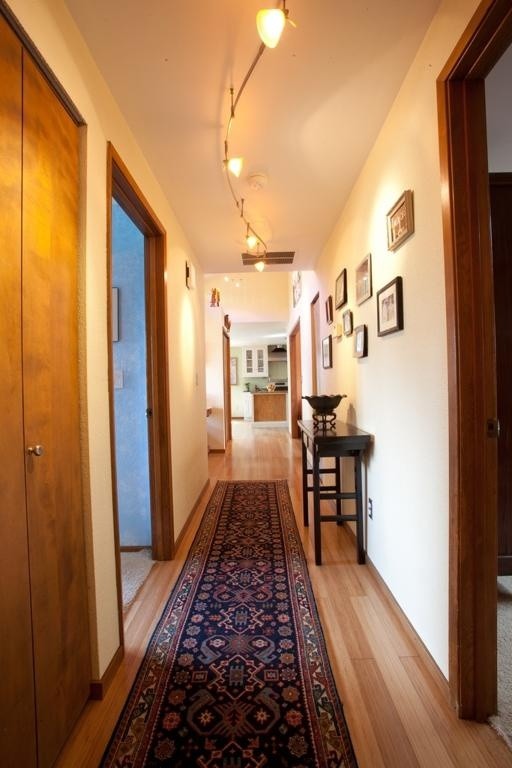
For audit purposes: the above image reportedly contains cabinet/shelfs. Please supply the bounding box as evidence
[242,344,269,378]
[254,394,286,421]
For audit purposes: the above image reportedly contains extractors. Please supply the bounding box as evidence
[268,345,287,361]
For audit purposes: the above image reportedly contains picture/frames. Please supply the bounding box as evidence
[321,333,332,369]
[353,324,367,359]
[385,189,413,250]
[334,268,347,308]
[375,275,404,337]
[325,296,332,325]
[111,287,120,342]
[343,307,352,337]
[356,249,372,307]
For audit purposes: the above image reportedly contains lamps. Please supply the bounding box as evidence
[221,2,293,273]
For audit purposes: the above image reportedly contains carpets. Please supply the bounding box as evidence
[97,479,361,766]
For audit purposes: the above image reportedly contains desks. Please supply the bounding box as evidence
[294,417,374,566]
